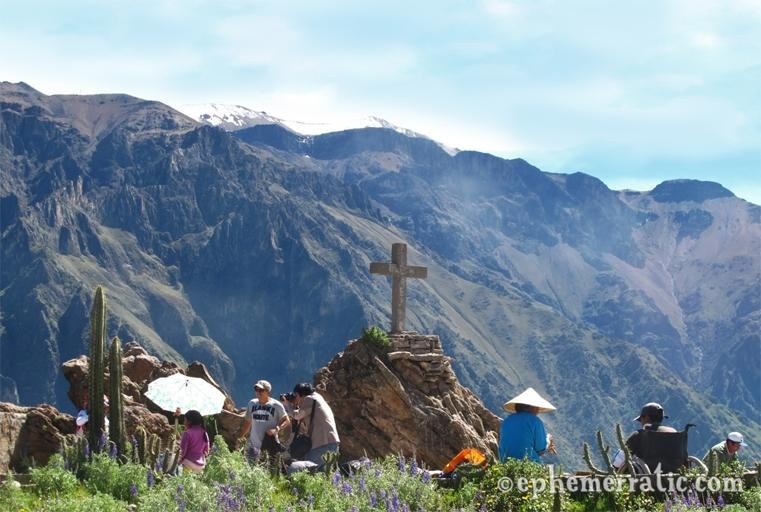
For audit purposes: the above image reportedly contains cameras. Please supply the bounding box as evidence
[279,392,295,402]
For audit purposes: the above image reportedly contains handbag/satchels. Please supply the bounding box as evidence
[288,436,313,458]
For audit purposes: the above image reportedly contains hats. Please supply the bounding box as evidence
[503,387,556,413]
[632,402,664,421]
[253,380,272,393]
[727,431,748,447]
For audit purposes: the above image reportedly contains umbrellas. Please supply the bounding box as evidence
[143,369,227,419]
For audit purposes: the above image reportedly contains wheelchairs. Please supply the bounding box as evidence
[616,422,710,475]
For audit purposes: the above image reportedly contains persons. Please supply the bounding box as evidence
[280,382,341,477]
[180,410,209,476]
[699,430,750,476]
[234,378,290,484]
[610,401,679,472]
[496,386,558,466]
